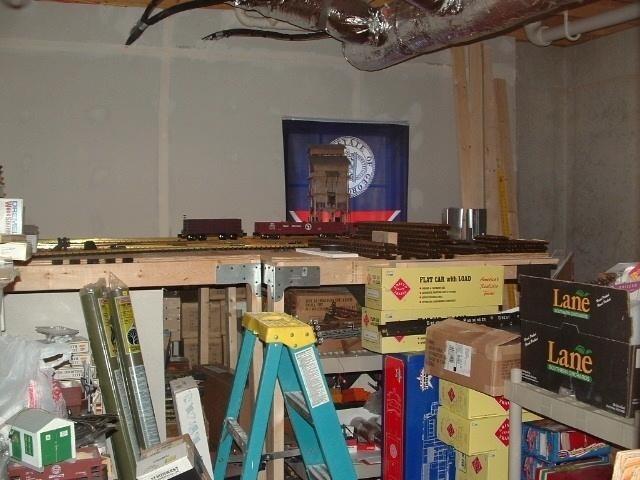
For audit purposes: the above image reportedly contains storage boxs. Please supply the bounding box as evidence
[364,267,506,311]
[381,353,455,480]
[362,307,517,355]
[423,318,521,396]
[439,378,530,419]
[437,405,544,456]
[287,289,363,351]
[455,449,509,480]
[518,272,640,418]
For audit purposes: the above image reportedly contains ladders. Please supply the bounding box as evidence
[214,312,357,480]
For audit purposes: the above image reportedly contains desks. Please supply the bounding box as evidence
[0,253,269,480]
[261,248,560,480]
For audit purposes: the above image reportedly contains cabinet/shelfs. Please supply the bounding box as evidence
[503,369,640,480]
[287,348,383,480]
